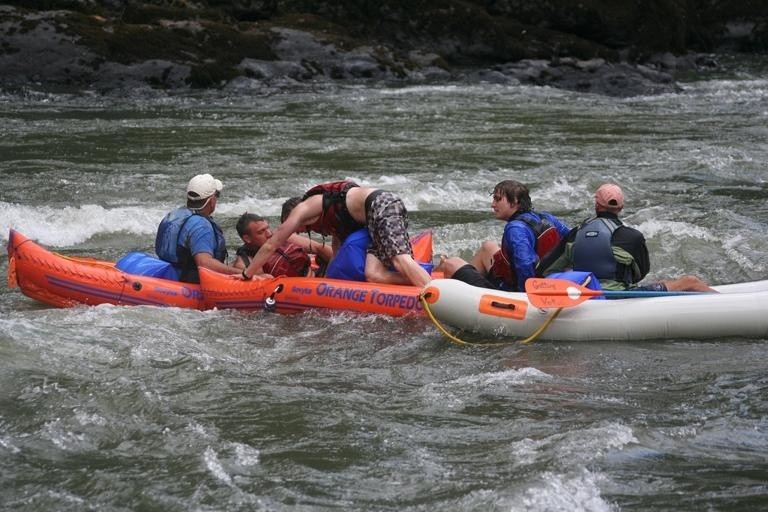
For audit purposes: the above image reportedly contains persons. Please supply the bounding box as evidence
[233,214,334,280]
[534,184,720,300]
[155,174,265,285]
[430,179,571,293]
[227,180,447,290]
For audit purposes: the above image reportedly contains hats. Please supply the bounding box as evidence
[187,174,222,201]
[594,184,624,209]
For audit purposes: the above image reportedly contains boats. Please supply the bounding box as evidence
[421,277,768,345]
[5,228,436,311]
[197,263,448,319]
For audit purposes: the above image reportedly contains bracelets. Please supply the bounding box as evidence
[242,269,253,280]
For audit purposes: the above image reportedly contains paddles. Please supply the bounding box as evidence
[524,278,706,309]
